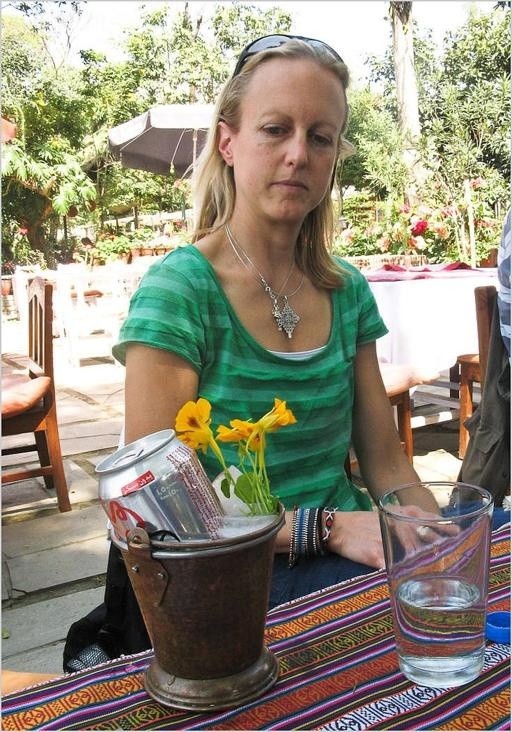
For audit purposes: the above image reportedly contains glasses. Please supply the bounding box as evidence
[233,34,345,78]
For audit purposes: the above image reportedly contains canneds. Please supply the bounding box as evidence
[94,428,228,541]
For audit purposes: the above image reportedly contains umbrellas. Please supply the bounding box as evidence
[106,102,216,239]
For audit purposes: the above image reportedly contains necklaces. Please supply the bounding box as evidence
[221,220,308,338]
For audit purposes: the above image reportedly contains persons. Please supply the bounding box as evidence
[497,208,511,360]
[113,32,462,655]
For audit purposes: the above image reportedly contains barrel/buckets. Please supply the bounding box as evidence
[107,496,287,713]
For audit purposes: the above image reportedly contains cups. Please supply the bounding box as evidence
[379,480,496,689]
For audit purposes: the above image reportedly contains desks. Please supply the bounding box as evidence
[0,514,509,730]
[350,267,501,393]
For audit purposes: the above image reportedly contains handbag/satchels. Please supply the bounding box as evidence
[63,543,153,675]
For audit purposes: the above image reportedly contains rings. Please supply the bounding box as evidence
[415,523,430,539]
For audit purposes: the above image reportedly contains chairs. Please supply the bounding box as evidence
[0,275,74,513]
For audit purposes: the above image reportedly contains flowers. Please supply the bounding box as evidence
[174,385,298,516]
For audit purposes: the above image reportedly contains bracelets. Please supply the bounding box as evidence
[287,503,339,569]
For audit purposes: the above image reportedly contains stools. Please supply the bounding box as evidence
[345,363,416,484]
